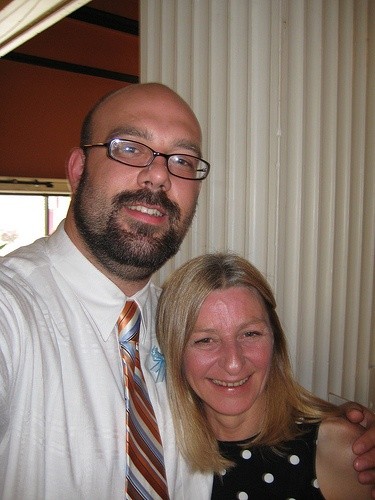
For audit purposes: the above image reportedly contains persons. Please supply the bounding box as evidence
[0,82,375,500]
[155,254,375,500]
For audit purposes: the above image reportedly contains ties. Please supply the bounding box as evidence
[118,300,170,500]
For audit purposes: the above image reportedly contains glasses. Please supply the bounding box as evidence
[83,138,211,180]
